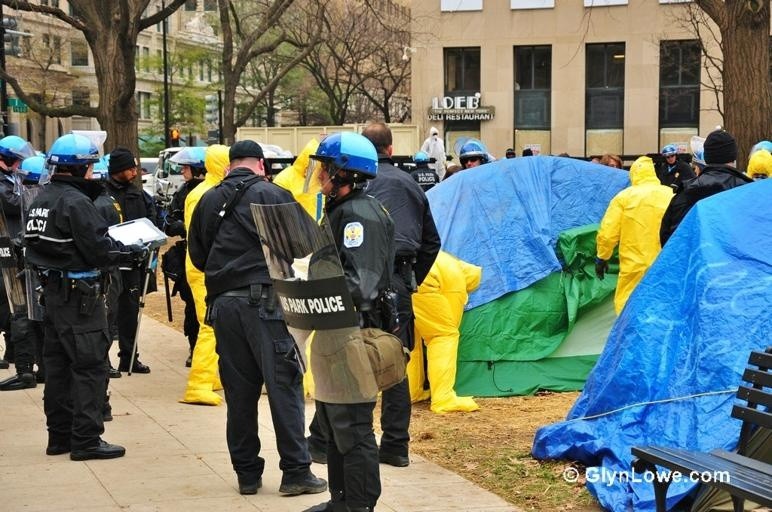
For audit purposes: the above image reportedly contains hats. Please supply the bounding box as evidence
[230,140,269,174]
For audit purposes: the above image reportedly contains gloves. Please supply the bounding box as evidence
[119,245,143,266]
[595,258,608,280]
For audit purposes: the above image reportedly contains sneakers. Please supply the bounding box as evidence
[238,439,408,512]
[103,352,150,422]
[46,438,70,455]
[0,356,44,389]
[70,437,125,460]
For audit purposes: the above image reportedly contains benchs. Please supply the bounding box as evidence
[628,349,768,511]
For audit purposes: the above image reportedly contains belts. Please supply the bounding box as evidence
[218,289,271,297]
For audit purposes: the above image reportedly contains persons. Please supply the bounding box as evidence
[303,120,440,467]
[304,131,395,512]
[187,141,328,494]
[22,129,143,460]
[0,131,228,406]
[404,126,772,415]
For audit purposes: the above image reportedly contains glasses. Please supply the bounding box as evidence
[462,158,481,164]
[667,155,675,158]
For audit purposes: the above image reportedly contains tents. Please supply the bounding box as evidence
[528,175,772,511]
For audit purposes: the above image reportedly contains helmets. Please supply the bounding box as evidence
[460,141,485,158]
[662,137,771,162]
[309,131,379,178]
[0,130,136,184]
[168,147,205,170]
[413,151,430,162]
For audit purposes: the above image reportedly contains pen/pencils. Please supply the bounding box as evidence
[116,221,135,227]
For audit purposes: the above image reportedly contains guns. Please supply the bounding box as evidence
[75,279,101,316]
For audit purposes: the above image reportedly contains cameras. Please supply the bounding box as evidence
[155,170,173,197]
